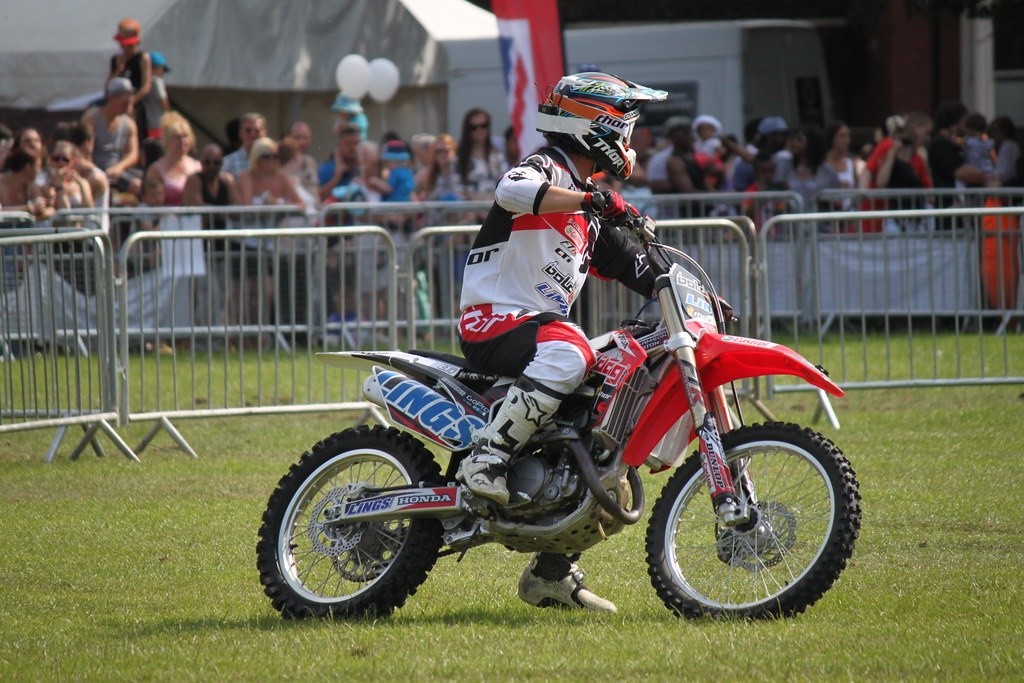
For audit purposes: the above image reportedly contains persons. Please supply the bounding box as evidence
[456,72,734,614]
[0,19,520,355]
[619,101,1024,308]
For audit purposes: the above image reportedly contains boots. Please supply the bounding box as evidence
[460,377,562,505]
[518,552,618,615]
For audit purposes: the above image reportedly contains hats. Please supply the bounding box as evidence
[664,105,695,135]
[114,19,140,46]
[690,114,723,140]
[754,117,788,141]
[150,52,171,72]
[107,77,137,97]
[330,92,364,114]
[379,140,411,161]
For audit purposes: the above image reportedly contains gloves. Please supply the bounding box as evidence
[580,188,626,224]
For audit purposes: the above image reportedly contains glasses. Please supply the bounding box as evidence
[52,156,70,162]
[260,153,279,159]
[245,127,260,134]
[469,122,487,130]
[202,159,223,166]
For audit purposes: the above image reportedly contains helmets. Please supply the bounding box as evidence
[535,71,669,182]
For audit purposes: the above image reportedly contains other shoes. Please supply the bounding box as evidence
[153,339,173,356]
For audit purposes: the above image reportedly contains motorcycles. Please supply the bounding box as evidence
[257,192,864,624]
